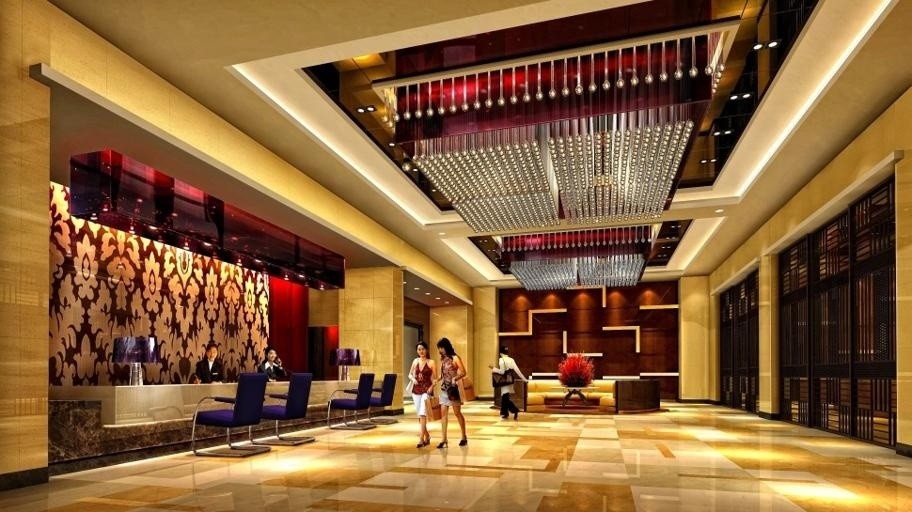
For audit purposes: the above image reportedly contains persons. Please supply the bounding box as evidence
[257,346,287,382]
[488,346,528,420]
[408,337,467,448]
[195,343,224,384]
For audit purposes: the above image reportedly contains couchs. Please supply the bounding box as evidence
[491,379,662,413]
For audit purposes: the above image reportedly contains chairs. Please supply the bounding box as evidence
[327,374,376,430]
[248,373,315,448]
[368,374,398,425]
[191,372,272,458]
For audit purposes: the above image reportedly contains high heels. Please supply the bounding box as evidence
[502,413,509,418]
[514,410,519,420]
[459,436,467,446]
[416,438,430,448]
[436,440,448,448]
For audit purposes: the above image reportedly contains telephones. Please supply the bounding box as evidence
[275,356,280,364]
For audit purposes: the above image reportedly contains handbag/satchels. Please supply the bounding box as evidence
[492,369,514,388]
[447,378,475,402]
[406,381,413,393]
[424,396,441,422]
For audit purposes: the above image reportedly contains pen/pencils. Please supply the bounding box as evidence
[194,374,199,384]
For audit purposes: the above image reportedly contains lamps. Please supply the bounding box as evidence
[328,347,361,380]
[111,335,158,385]
